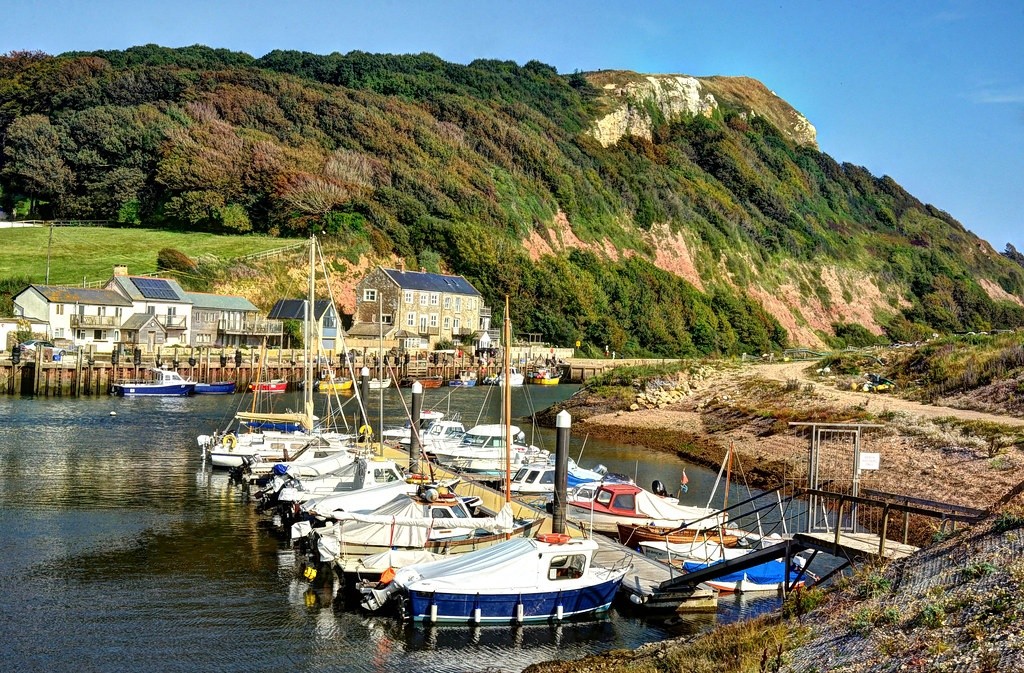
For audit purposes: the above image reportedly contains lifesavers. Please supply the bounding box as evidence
[439,493,454,498]
[413,474,429,480]
[406,478,429,484]
[222,435,236,449]
[536,533,571,544]
[358,425,372,439]
[651,480,665,495]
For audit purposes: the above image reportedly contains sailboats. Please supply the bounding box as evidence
[196,234,819,633]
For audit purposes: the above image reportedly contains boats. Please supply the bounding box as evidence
[191,380,236,394]
[110,367,197,397]
